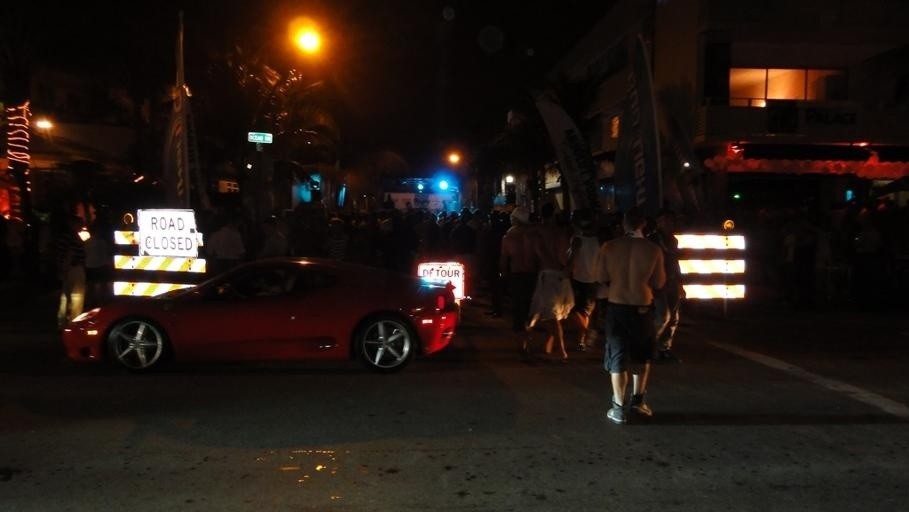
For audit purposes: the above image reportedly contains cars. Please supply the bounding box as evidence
[62,255,461,376]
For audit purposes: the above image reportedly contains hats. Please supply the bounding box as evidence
[512,207,529,225]
[570,208,592,232]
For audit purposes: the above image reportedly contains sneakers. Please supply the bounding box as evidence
[606,397,628,424]
[629,390,653,417]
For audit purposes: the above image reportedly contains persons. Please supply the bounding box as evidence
[1,208,186,337]
[200,202,480,300]
[481,200,684,424]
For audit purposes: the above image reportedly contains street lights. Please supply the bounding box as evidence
[252,24,322,259]
